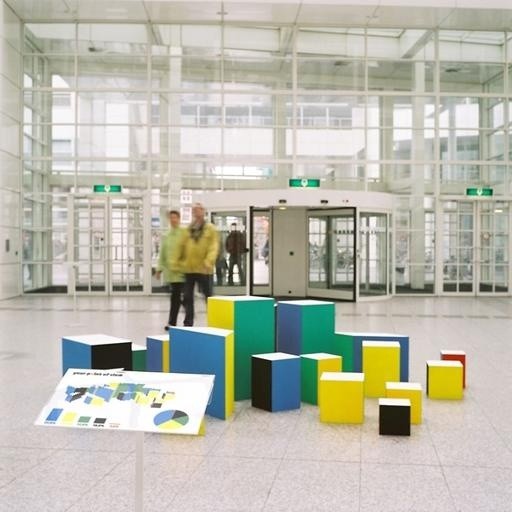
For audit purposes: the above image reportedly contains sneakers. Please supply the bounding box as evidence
[165,326,176,330]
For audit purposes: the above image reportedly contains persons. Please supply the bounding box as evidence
[154,200,245,334]
